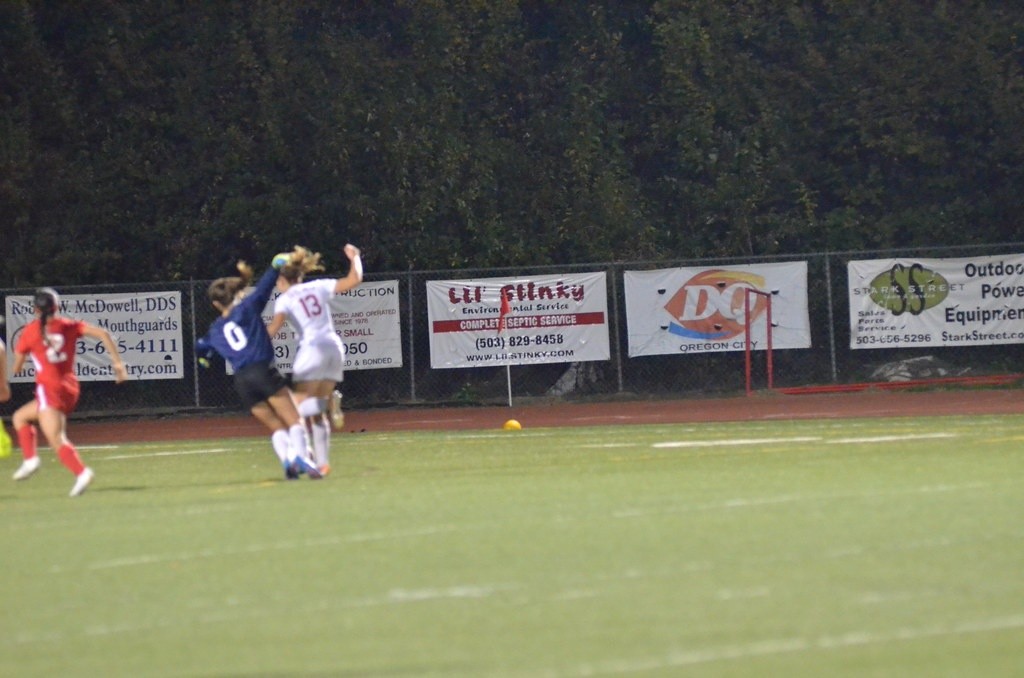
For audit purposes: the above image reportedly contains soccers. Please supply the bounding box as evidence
[503,419,522,430]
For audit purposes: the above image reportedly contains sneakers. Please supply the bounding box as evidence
[13,456,40,482]
[330,390,345,429]
[282,452,330,480]
[69,467,94,497]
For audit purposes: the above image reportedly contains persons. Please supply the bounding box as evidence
[266,244,363,475]
[196,267,323,480]
[0,336,12,402]
[10,287,126,495]
